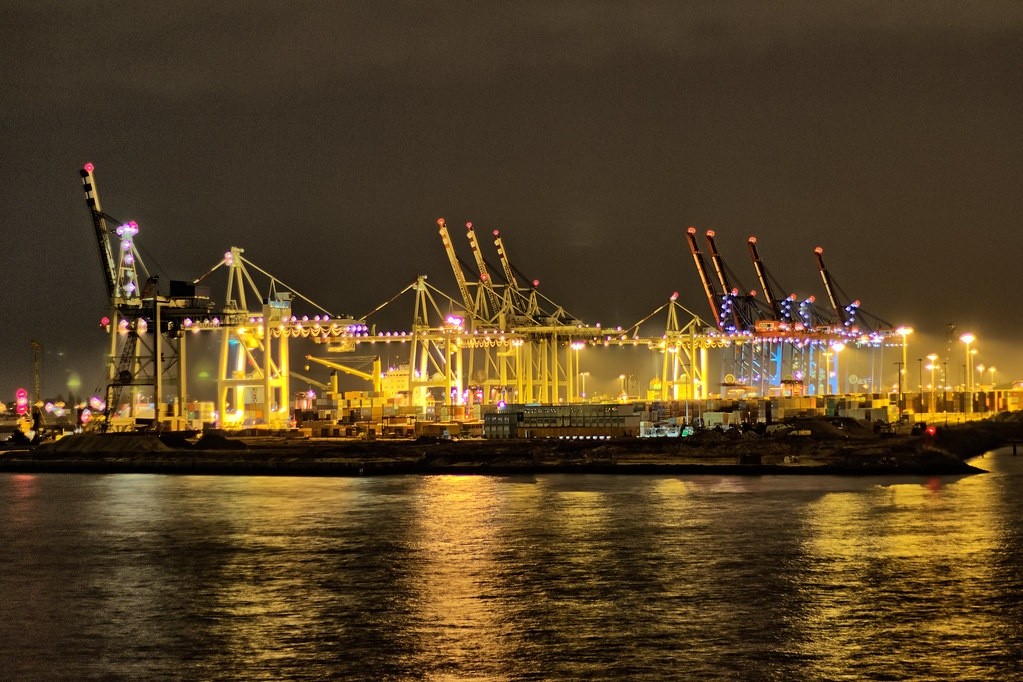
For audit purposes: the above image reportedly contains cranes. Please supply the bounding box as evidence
[678,226,899,424]
[383,216,631,433]
[77,160,217,433]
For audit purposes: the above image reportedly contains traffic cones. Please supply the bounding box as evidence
[989,367,998,391]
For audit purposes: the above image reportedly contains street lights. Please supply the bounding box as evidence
[976,364,987,390]
[927,352,939,417]
[961,332,976,413]
[571,344,584,403]
[578,372,590,401]
[894,327,915,396]
[619,374,628,401]
[968,348,979,392]
